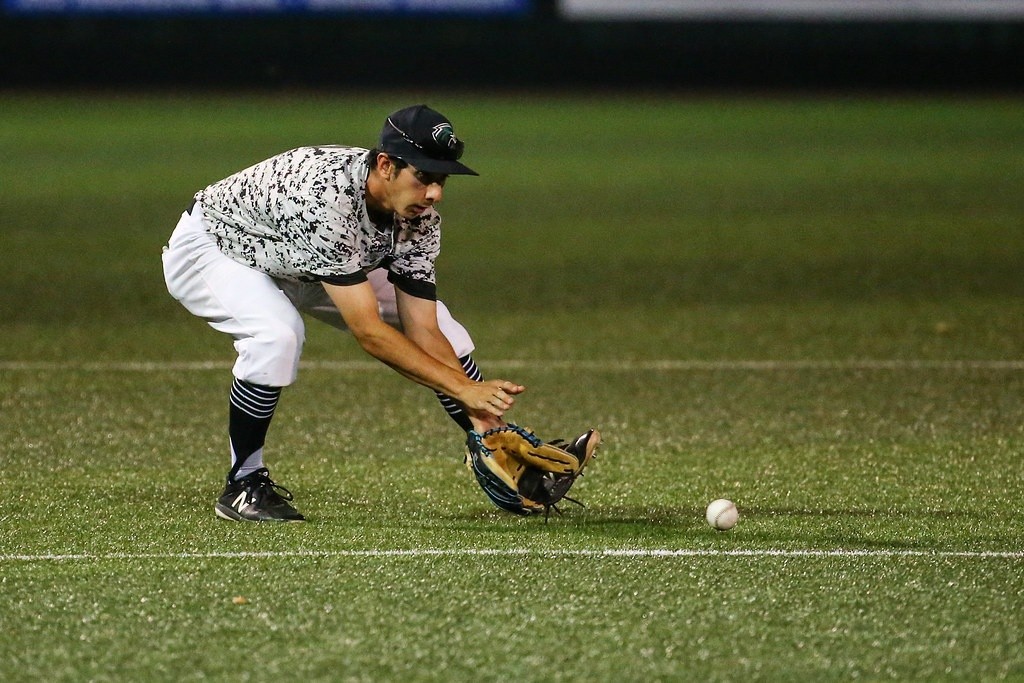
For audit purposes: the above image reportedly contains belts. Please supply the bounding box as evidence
[187,199,196,214]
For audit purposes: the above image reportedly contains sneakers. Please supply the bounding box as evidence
[215,467,306,524]
[548,428,602,481]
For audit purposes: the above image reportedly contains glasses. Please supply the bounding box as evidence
[388,117,463,160]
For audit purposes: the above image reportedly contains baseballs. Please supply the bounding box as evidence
[707,499,739,530]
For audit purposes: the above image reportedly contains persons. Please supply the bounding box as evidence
[162,105,602,525]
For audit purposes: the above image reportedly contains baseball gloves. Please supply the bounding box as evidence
[465,420,601,516]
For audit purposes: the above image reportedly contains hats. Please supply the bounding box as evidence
[377,104,479,176]
